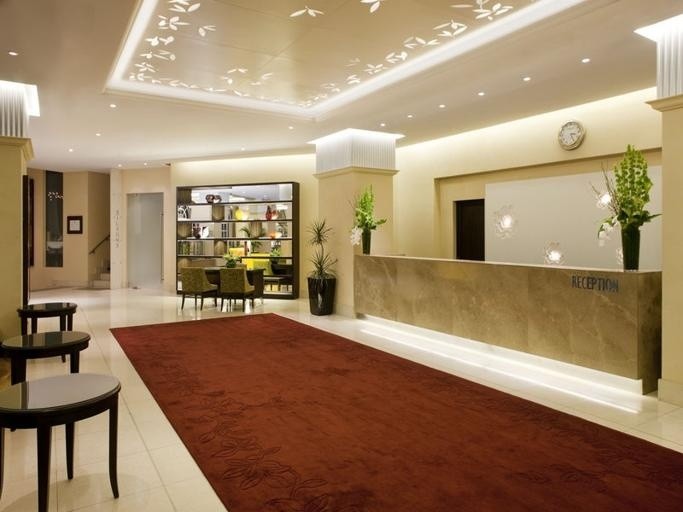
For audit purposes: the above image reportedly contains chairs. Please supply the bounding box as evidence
[180,267,256,312]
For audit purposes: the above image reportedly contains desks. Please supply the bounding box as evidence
[191,267,266,305]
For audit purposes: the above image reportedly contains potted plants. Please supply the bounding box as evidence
[305,219,337,315]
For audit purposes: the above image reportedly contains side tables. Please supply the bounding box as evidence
[1,303,125,512]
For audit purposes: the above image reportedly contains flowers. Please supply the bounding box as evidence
[592,142,664,246]
[348,183,388,246]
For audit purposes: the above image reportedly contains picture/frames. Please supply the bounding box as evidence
[66,215,83,234]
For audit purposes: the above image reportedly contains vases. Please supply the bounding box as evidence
[621,226,641,270]
[360,226,373,254]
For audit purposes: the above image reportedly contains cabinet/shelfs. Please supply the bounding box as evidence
[175,180,299,300]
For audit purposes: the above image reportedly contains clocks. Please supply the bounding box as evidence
[557,118,584,149]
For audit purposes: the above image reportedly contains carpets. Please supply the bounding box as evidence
[107,312,682,512]
